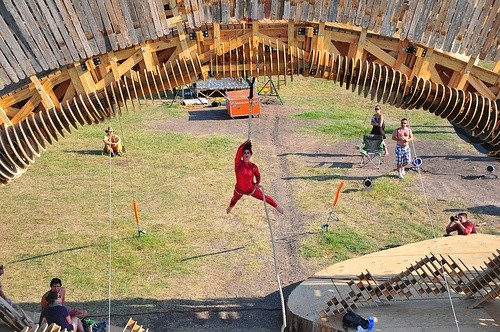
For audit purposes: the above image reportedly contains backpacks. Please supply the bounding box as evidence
[342,310,369,330]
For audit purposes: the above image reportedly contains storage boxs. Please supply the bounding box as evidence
[226,89,261,117]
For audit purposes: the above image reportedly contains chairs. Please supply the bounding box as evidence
[356,134,385,169]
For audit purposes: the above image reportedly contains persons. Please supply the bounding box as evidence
[226,139,285,215]
[37,292,85,332]
[370,105,389,158]
[446,213,477,236]
[102,126,124,157]
[0,265,13,319]
[39,278,88,323]
[392,117,414,179]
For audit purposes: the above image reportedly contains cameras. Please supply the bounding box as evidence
[450,216,459,222]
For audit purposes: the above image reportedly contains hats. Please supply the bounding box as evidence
[105,127,114,133]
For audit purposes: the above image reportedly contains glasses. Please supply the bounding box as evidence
[401,123,407,124]
[57,295,61,298]
[375,108,380,110]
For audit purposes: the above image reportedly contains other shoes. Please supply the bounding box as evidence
[382,151,389,156]
[111,153,115,156]
[118,153,122,156]
[397,173,403,179]
[401,166,405,175]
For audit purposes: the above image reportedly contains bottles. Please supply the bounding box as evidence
[368,315,374,332]
